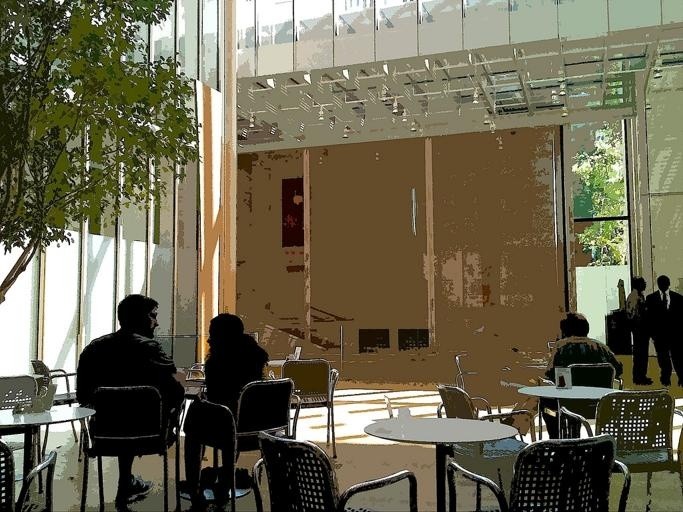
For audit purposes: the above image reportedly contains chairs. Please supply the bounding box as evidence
[0,337,683,512]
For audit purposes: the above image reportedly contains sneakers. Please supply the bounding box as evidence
[128,479,154,501]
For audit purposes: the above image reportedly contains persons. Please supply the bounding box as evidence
[173,312,269,511]
[625,274,653,385]
[541,311,624,439]
[645,274,682,387]
[74,293,183,504]
